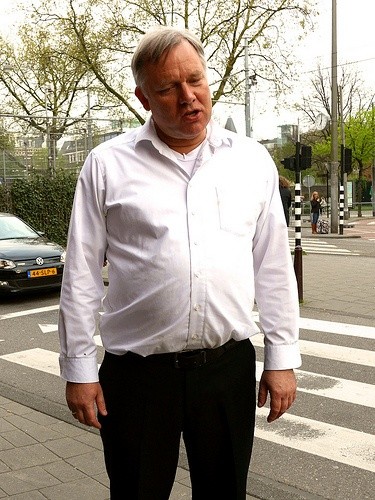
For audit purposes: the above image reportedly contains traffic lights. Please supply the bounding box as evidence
[280,157,296,171]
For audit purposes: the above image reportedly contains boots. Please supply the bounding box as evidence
[311,223,318,234]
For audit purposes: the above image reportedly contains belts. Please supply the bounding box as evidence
[126,339,240,371]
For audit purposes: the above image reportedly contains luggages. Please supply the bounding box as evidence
[318,207,330,234]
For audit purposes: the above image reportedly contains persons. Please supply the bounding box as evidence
[57,26,302,500]
[310,191,323,234]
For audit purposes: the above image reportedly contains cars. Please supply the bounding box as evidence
[0,212,67,300]
